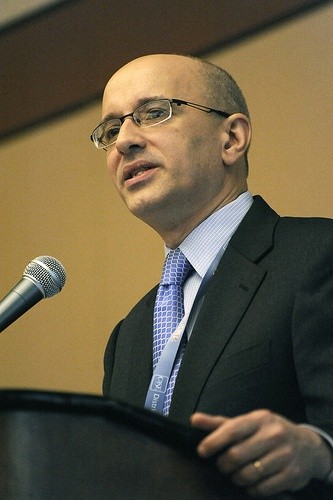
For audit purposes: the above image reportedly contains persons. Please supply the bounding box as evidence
[92,52,333,500]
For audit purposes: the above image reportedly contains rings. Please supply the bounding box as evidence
[252,460,267,478]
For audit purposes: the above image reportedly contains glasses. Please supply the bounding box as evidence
[89,98,232,150]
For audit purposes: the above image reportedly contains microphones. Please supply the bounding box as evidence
[0,255,66,331]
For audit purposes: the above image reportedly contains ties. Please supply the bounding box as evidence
[152,248,193,418]
